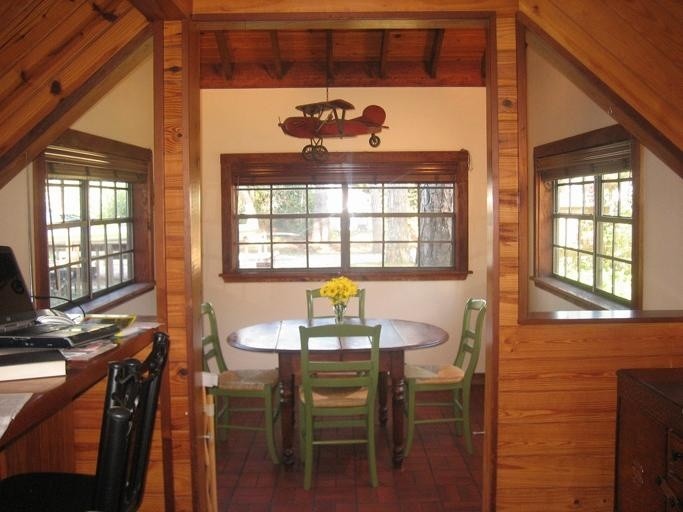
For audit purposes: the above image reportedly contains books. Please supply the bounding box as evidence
[0,349,70,382]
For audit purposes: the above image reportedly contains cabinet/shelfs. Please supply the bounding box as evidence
[613,367,683,512]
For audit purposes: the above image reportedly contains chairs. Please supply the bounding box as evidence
[0,332,171,512]
[404,297,486,455]
[198,302,283,466]
[304,288,366,318]
[294,324,382,490]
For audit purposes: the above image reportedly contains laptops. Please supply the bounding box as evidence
[0,245,120,348]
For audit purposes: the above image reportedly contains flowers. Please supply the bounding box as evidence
[319,276,360,307]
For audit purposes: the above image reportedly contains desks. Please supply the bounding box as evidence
[0,318,165,450]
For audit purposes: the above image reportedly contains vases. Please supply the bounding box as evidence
[333,307,345,324]
